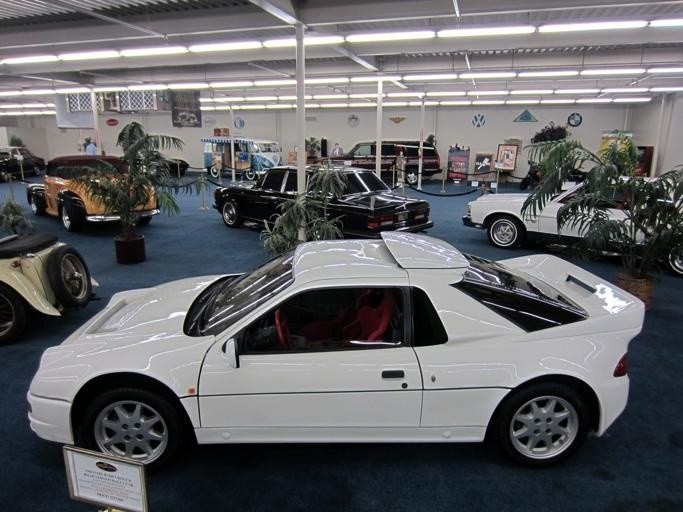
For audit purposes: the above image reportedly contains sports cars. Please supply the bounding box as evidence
[23,233,649,467]
[120,150,189,178]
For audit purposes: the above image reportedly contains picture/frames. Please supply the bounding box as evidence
[497,142,518,170]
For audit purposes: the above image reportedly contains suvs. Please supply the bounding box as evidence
[310,139,442,186]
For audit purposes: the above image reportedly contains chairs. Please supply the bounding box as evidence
[339,299,391,346]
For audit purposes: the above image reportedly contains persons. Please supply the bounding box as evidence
[330,142,343,156]
[86,139,96,155]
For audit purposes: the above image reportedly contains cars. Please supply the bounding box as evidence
[0,146,45,181]
[25,155,161,232]
[212,164,436,242]
[458,175,682,279]
[0,231,99,344]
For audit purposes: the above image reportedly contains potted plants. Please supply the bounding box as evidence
[68,118,203,264]
[522,126,681,311]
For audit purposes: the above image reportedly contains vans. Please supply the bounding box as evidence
[203,138,282,180]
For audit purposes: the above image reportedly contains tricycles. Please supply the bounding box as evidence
[200,136,256,180]
